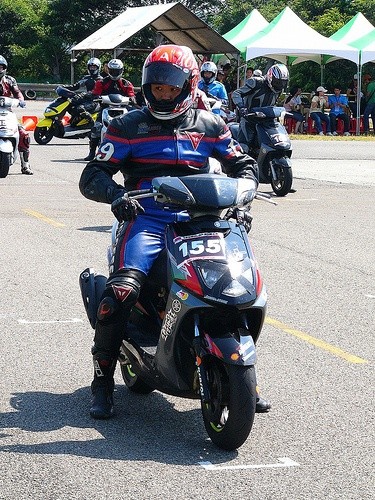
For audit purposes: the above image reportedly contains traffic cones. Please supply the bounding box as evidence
[21,115,38,133]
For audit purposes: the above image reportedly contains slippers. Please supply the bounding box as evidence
[302,130,307,134]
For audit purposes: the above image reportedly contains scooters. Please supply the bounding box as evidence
[34,85,98,145]
[80,173,281,454]
[0,95,26,178]
[94,93,138,151]
[226,105,293,198]
[194,90,235,123]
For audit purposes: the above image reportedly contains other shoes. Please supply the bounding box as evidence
[325,131,333,136]
[333,131,339,136]
[360,132,370,136]
[343,132,351,135]
[319,131,325,135]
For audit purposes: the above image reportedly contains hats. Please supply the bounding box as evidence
[363,75,371,81]
[317,86,328,93]
[353,74,357,81]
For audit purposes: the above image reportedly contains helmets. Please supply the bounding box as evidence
[266,63,289,94]
[86,57,101,77]
[141,45,201,120]
[200,61,218,84]
[107,59,124,80]
[0,55,7,78]
[253,69,262,77]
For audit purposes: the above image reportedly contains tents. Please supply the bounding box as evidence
[212,6,375,137]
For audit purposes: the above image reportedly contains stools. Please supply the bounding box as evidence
[283,117,297,133]
[308,117,326,134]
[348,118,357,134]
[360,117,364,134]
[337,117,344,134]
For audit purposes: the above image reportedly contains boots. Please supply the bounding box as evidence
[255,395,270,412]
[84,140,98,161]
[89,350,117,418]
[19,150,34,175]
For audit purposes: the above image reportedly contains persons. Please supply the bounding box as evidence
[77,45,270,418]
[0,55,375,193]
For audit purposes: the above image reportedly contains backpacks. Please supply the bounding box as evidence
[301,97,311,108]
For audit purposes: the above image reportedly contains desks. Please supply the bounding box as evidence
[299,108,331,133]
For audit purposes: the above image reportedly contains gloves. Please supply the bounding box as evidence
[74,91,88,103]
[94,95,102,103]
[63,81,80,91]
[286,111,292,118]
[19,101,26,107]
[110,197,145,223]
[129,97,137,105]
[224,204,253,234]
[237,101,249,117]
